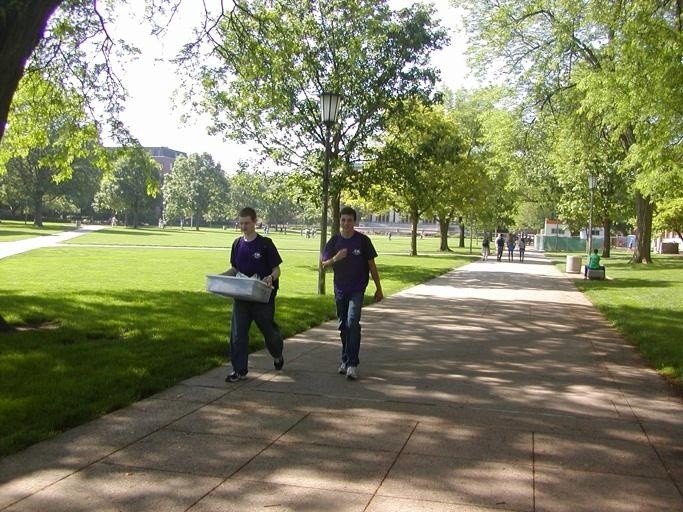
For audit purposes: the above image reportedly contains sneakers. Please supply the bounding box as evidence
[225,370,248,383]
[273,356,284,370]
[337,360,361,380]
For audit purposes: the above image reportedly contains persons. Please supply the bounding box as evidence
[321,207,383,380]
[217,208,284,382]
[386,232,391,240]
[111,215,118,228]
[159,217,167,229]
[262,224,269,234]
[302,227,317,239]
[481,233,525,261]
[585,248,605,279]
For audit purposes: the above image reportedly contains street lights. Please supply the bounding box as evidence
[317,92,343,295]
[555,210,558,253]
[588,176,597,265]
[469,196,477,253]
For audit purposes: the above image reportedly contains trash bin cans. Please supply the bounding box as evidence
[566,255,581,273]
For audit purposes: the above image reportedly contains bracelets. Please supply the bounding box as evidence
[332,256,337,264]
[269,275,274,281]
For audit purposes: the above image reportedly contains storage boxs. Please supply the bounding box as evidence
[206,274,274,304]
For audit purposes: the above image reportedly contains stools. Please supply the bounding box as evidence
[588,269,604,280]
